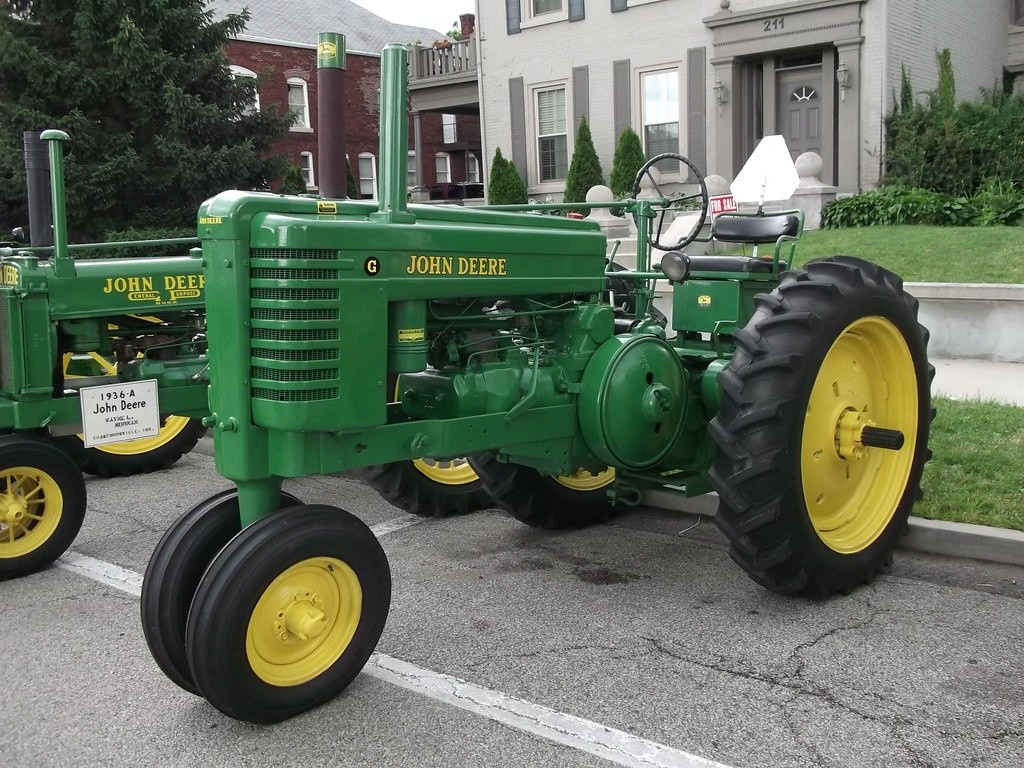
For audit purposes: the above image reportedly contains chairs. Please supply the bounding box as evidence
[671,209,805,349]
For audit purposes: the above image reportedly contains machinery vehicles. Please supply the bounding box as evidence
[141,31,936,728]
[1,128,492,582]
